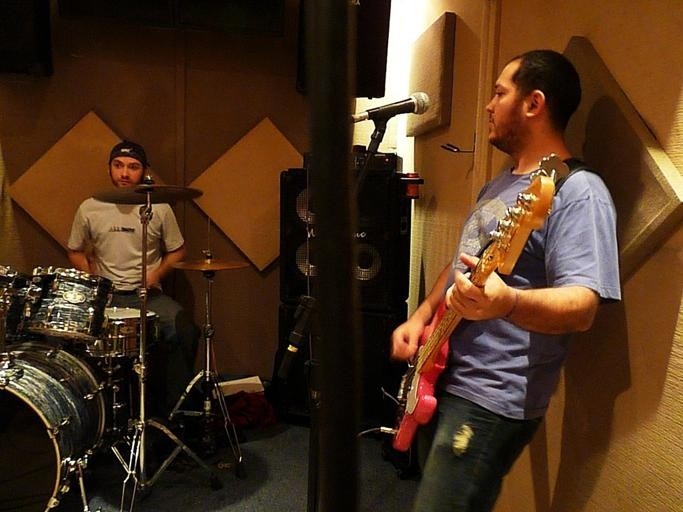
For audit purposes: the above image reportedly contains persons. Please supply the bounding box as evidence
[390,48,623,511]
[66,137,215,464]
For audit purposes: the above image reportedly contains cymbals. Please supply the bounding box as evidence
[175,258,251,271]
[93,183,202,204]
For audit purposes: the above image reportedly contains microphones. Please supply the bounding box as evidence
[352,92,430,122]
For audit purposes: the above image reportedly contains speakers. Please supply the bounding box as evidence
[0,0,55,74]
[296,0,390,98]
[279,168,411,420]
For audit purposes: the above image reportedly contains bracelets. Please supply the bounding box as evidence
[505,289,518,318]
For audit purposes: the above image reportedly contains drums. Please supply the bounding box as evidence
[16,267,114,340]
[0,345,106,510]
[80,305,158,359]
[0,266,32,345]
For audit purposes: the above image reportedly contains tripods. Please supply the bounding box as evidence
[112,191,223,512]
[148,274,246,479]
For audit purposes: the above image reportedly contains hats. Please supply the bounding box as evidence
[109,142,147,167]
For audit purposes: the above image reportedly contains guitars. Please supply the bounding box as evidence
[391,170,554,454]
